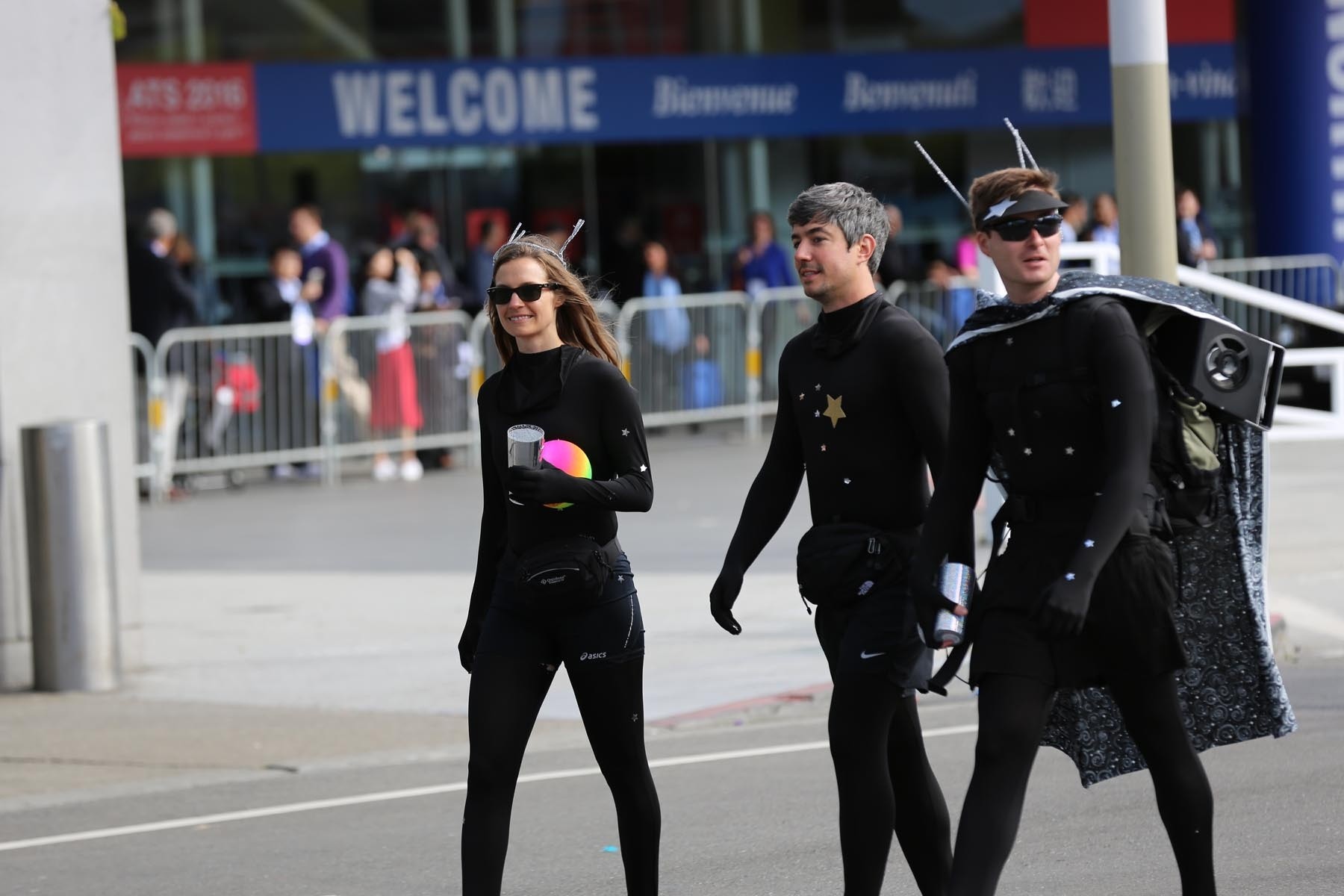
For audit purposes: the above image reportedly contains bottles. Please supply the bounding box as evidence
[934,561,975,644]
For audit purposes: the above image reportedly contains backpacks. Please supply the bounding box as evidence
[1123,302,1225,540]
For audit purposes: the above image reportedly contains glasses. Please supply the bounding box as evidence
[486,283,561,304]
[986,215,1063,242]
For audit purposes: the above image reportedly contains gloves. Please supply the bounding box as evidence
[1039,572,1094,638]
[458,620,479,673]
[710,566,742,635]
[506,458,577,504]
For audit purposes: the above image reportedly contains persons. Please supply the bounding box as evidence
[133,169,1222,490]
[459,230,661,896]
[708,183,965,896]
[939,168,1218,896]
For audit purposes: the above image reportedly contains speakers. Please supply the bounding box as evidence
[1156,316,1283,433]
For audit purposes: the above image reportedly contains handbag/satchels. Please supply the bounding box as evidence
[796,523,901,609]
[515,538,605,602]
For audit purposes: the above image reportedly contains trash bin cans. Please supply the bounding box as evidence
[20,419,122,693]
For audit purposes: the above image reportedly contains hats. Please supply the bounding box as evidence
[979,190,1069,227]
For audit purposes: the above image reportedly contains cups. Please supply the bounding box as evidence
[507,424,545,505]
